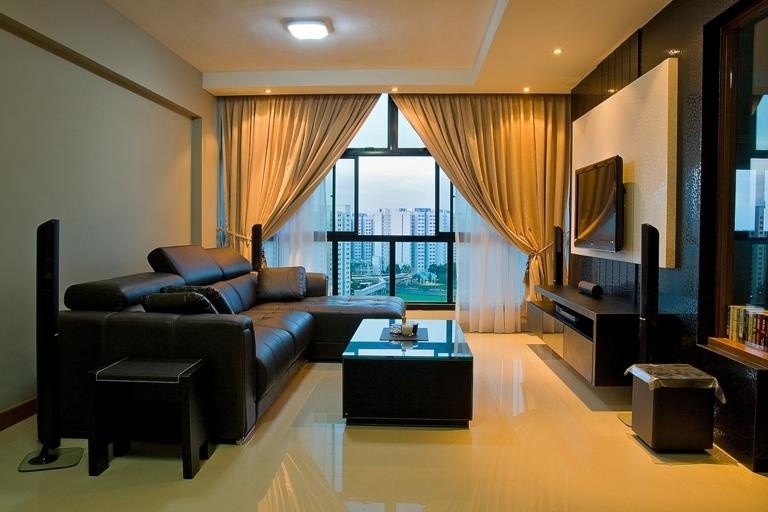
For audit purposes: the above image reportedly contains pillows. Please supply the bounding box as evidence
[258,266,307,302]
[141,284,235,318]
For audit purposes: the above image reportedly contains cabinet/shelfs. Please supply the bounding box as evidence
[527,284,670,390]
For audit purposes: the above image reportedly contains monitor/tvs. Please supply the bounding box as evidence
[573,156,626,253]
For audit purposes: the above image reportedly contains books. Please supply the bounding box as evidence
[725,302,768,353]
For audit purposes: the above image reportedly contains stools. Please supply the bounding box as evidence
[631,364,718,455]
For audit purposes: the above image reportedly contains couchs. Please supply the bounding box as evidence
[59,243,406,444]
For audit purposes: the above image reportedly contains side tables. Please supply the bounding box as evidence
[86,356,216,481]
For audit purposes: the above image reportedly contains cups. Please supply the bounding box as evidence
[388,322,419,337]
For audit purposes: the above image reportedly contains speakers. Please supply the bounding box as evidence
[553,226,563,288]
[640,223,659,366]
